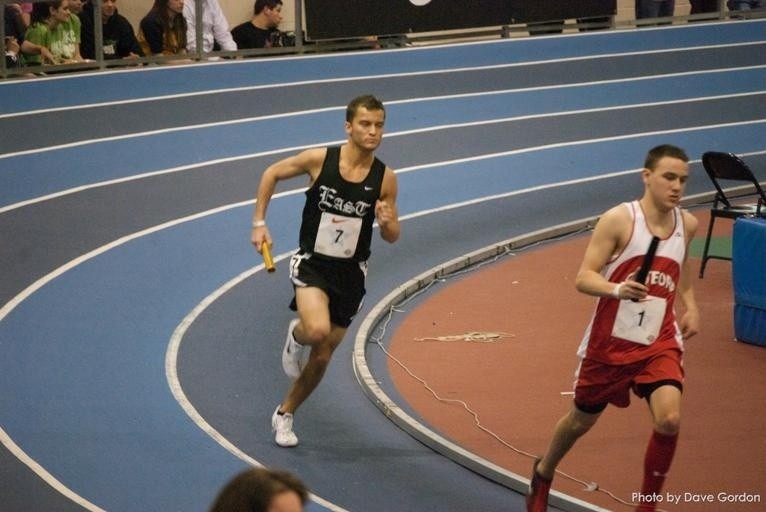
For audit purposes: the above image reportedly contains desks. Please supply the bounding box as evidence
[732,216,765,347]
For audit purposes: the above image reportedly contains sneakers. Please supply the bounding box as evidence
[271,405,300,448]
[282,318,305,382]
[526,457,552,512]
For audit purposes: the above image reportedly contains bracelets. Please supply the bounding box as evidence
[251,219,265,227]
[612,280,620,300]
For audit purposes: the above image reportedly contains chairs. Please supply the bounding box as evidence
[699,150,766,279]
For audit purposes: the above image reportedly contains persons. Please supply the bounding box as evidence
[2,3,98,79]
[138,0,200,66]
[215,0,297,60]
[202,467,310,512]
[182,0,243,64]
[79,1,150,67]
[531,143,701,508]
[306,0,766,54]
[250,94,401,445]
